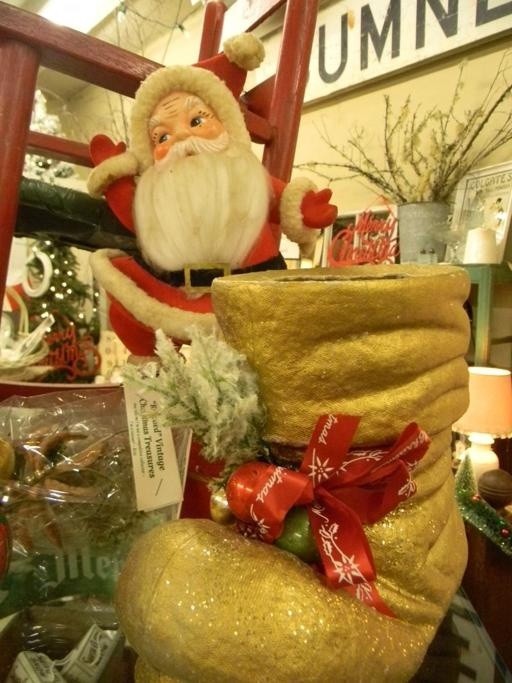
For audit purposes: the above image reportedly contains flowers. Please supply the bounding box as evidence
[308,45,512,203]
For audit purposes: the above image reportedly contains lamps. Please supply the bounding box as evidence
[113,1,187,35]
[451,365,512,497]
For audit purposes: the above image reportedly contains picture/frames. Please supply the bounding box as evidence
[445,159,512,264]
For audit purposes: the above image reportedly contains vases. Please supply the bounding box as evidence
[398,201,451,263]
